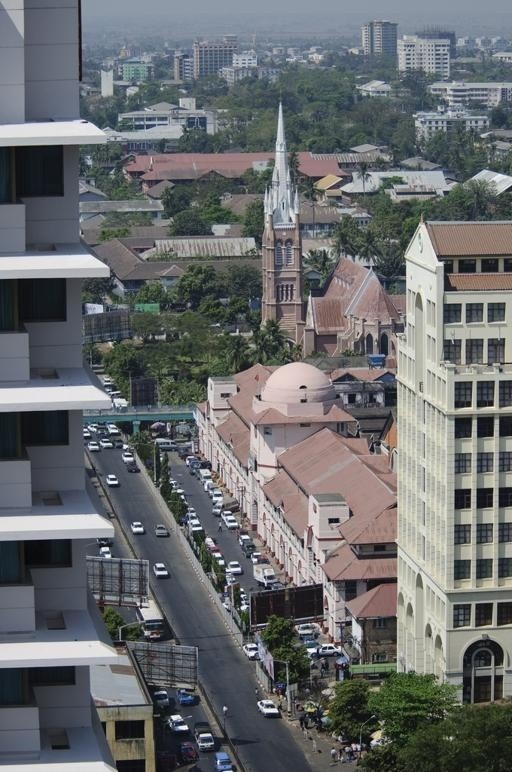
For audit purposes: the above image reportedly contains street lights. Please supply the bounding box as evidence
[260,659,292,718]
[308,657,324,699]
[222,704,229,740]
[358,714,376,765]
[163,715,192,750]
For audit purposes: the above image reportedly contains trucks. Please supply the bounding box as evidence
[194,721,215,752]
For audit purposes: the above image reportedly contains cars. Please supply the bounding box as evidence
[176,688,195,705]
[153,690,170,709]
[176,741,197,762]
[256,699,280,718]
[82,374,350,671]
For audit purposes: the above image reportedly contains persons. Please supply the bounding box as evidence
[218,520,222,532]
[254,663,366,763]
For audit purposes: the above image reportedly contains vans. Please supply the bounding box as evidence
[213,752,234,772]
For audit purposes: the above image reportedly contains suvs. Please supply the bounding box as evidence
[168,714,190,736]
